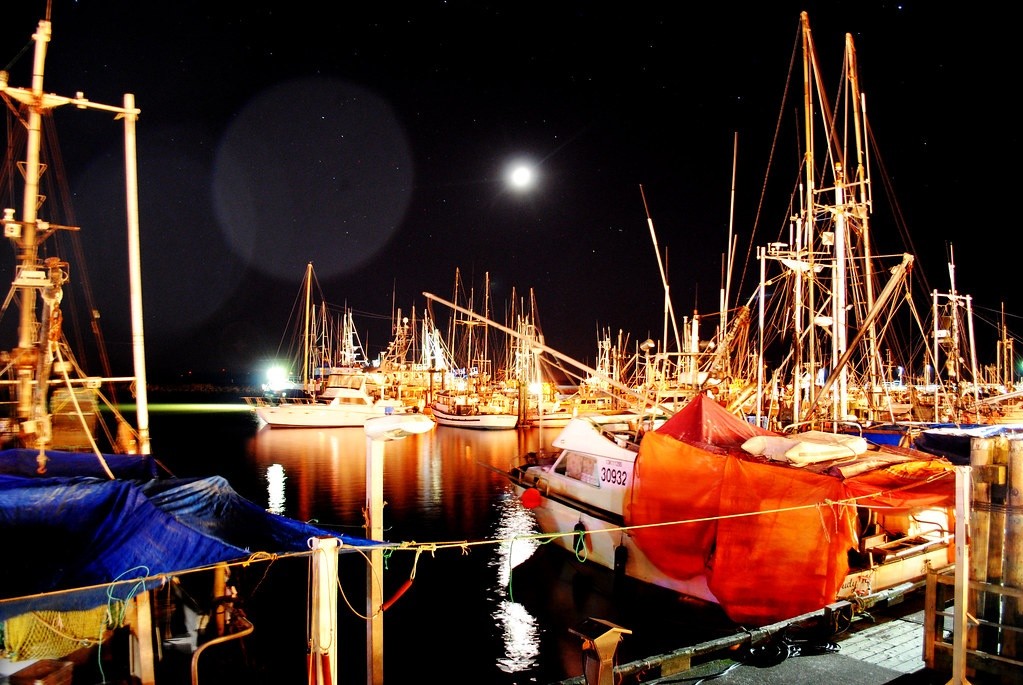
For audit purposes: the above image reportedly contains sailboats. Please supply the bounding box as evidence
[0,1,395,685]
[235,11,1023,475]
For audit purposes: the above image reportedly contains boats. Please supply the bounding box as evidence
[500,392,964,619]
